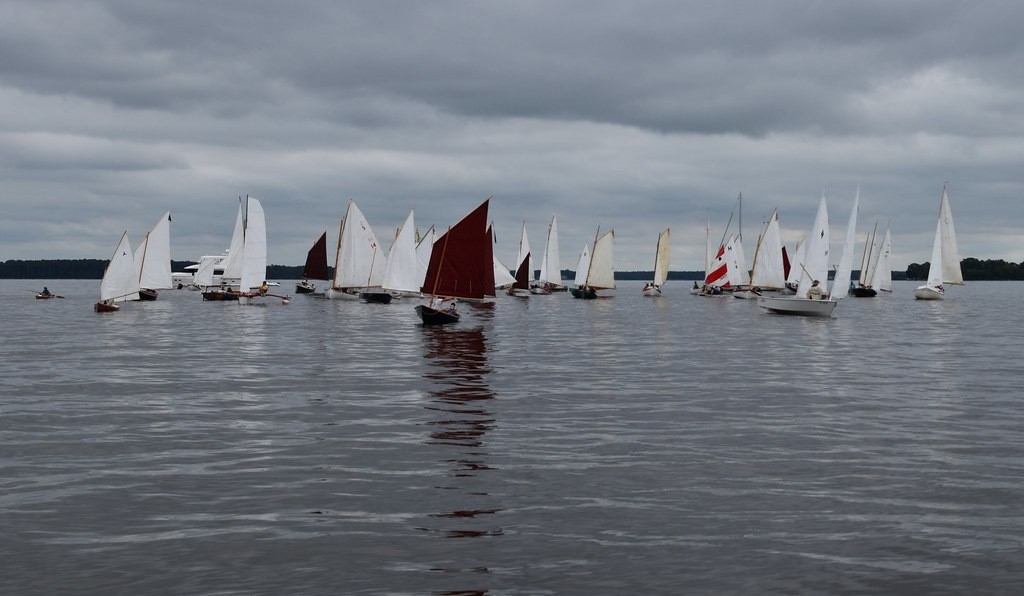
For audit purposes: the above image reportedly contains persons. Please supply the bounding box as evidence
[446,302,459,316]
[544,283,557,292]
[643,283,661,292]
[302,279,315,289]
[702,284,723,295]
[693,281,699,289]
[42,287,49,295]
[807,280,829,300]
[849,281,855,288]
[255,281,268,294]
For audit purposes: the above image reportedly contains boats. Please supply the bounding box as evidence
[35,293,65,299]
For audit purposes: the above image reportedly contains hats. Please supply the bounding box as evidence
[813,280,819,285]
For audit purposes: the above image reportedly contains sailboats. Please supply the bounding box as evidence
[687,191,856,301]
[571,224,620,299]
[415,196,493,326]
[127,210,174,302]
[754,187,838,317]
[94,228,142,312]
[332,196,568,304]
[642,227,676,296]
[914,182,965,300]
[295,230,329,295]
[192,195,268,301]
[851,220,892,297]
